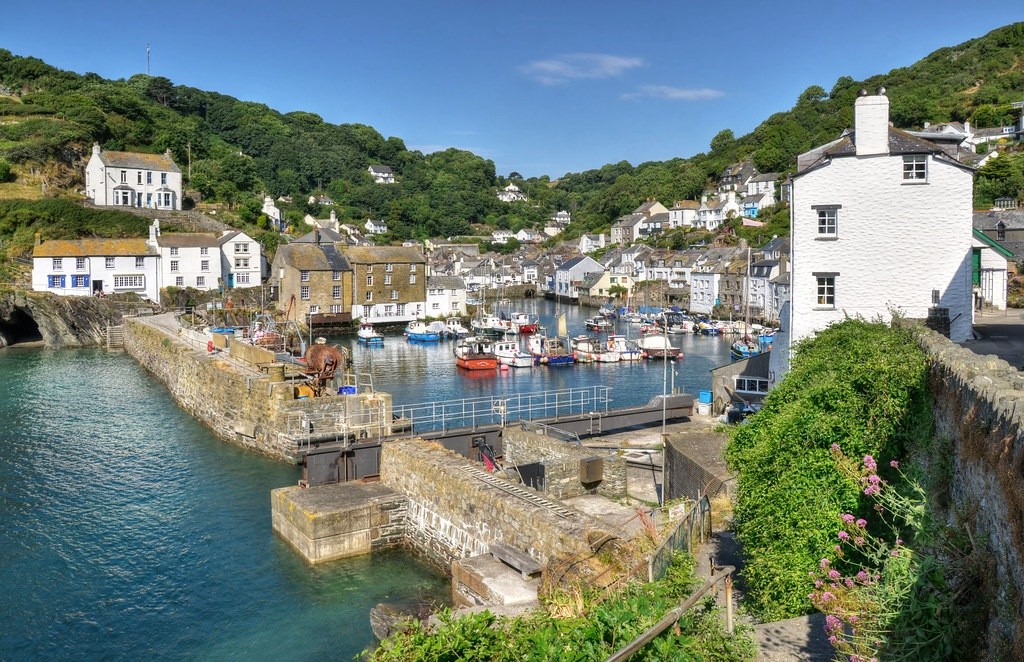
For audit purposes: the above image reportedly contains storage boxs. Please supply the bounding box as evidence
[699,389,714,403]
[698,403,713,415]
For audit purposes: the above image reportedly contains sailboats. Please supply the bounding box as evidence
[730,246,761,360]
[465,258,512,306]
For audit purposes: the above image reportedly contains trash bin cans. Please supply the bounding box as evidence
[698,390,713,403]
[338,387,356,395]
[212,326,235,335]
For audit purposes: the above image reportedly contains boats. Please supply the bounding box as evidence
[426,313,540,341]
[357,323,385,342]
[716,322,734,333]
[701,324,719,336]
[570,335,620,362]
[733,320,754,334]
[492,341,534,367]
[635,330,681,357]
[757,335,774,343]
[752,324,772,334]
[601,335,643,360]
[624,313,642,323]
[599,307,616,319]
[453,336,497,370]
[526,334,574,366]
[655,311,688,334]
[585,316,612,331]
[403,321,439,340]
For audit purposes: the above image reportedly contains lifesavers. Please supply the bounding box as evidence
[410,325,414,329]
[586,353,592,360]
